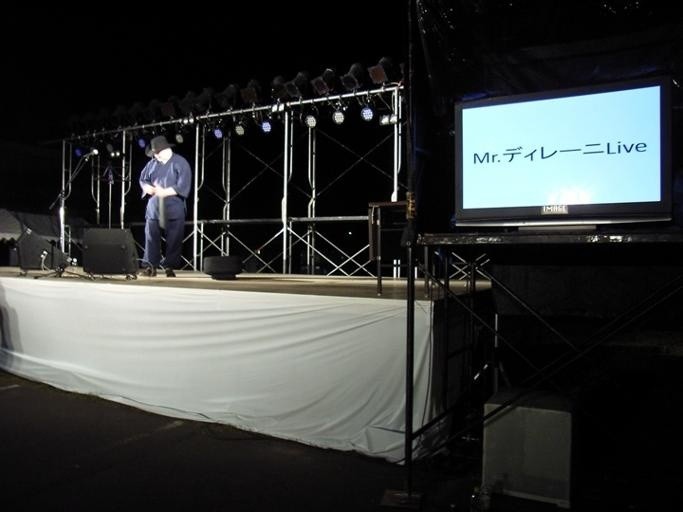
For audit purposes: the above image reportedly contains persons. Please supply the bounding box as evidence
[137,134,192,277]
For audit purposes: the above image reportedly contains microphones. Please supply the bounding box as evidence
[81,149,100,159]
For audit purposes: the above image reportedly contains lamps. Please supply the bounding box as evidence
[232,115,247,136]
[358,94,373,122]
[258,111,274,132]
[303,104,320,129]
[175,124,187,142]
[375,91,395,124]
[329,98,347,124]
[75,128,147,160]
[213,120,224,140]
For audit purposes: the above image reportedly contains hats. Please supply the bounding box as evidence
[145,136,175,157]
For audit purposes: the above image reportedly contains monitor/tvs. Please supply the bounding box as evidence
[456,76,671,227]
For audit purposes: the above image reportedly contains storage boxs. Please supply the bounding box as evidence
[480,391,579,510]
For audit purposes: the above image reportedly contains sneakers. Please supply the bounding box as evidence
[165,269,175,277]
[139,267,156,276]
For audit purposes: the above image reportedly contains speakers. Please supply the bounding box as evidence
[16,227,68,270]
[84,228,139,274]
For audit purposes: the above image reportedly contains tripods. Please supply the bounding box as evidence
[33,164,89,280]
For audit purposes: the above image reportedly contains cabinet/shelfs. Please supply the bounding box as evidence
[399,193,683,497]
